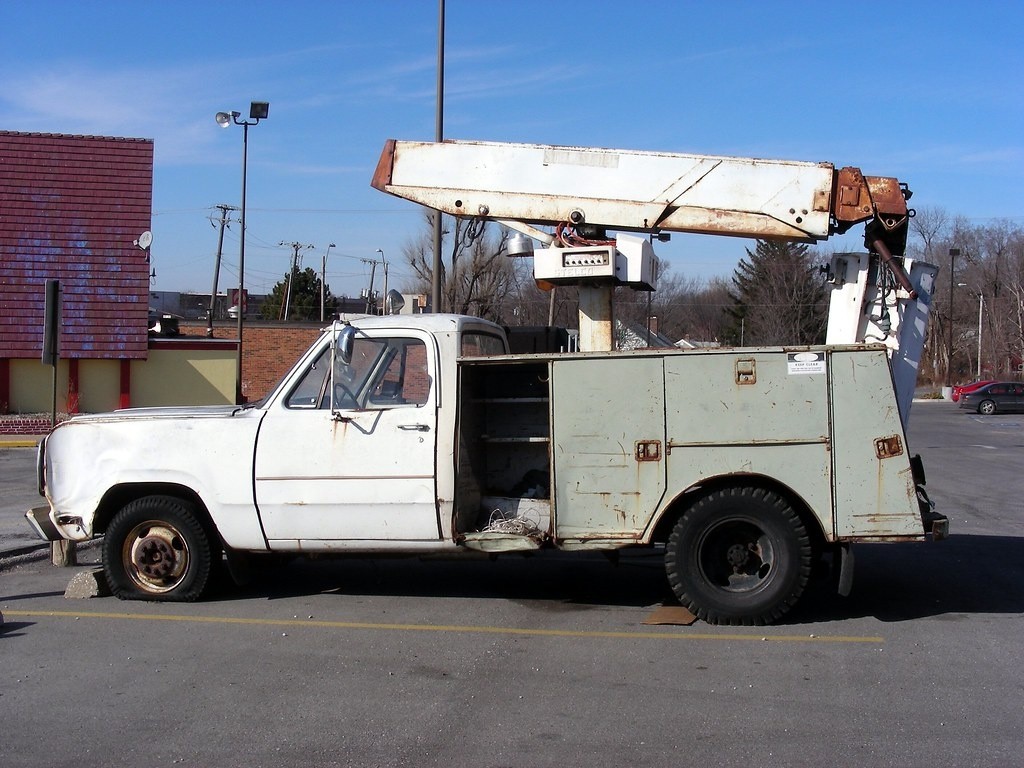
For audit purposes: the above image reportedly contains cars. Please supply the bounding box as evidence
[952,380,1022,402]
[958,381,1024,416]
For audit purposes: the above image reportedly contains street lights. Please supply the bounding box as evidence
[946,248,960,386]
[958,283,983,381]
[376,249,388,316]
[321,243,336,322]
[215,101,270,408]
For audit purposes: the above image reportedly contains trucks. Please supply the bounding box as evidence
[23,140,950,626]
